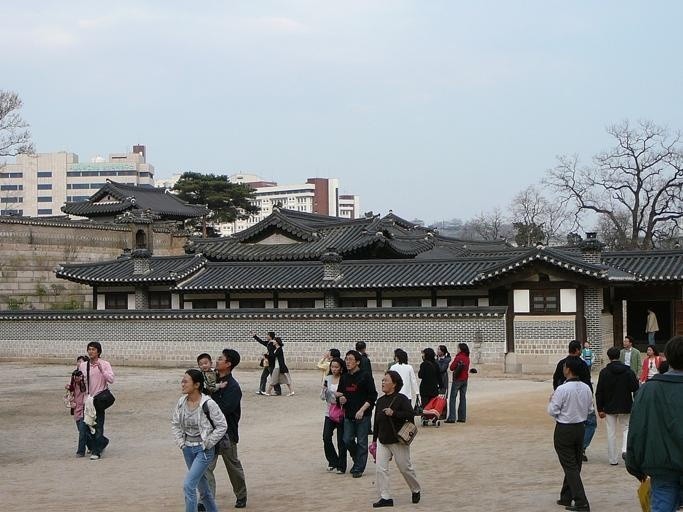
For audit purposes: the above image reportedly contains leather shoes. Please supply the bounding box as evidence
[412,491,420,503]
[565,502,590,511]
[198,503,204,511]
[457,420,466,422]
[444,420,455,423]
[255,390,295,397]
[556,499,571,506]
[373,498,393,507]
[235,499,246,508]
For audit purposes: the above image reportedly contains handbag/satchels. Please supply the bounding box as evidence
[328,402,344,424]
[202,399,233,455]
[388,394,418,446]
[94,389,116,411]
[414,395,424,416]
[320,370,327,401]
[422,393,445,418]
[263,356,270,367]
[453,360,465,378]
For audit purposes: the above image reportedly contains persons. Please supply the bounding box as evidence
[645,308,659,346]
[172,332,295,512]
[64,356,109,454]
[549,336,683,511]
[317,342,471,508]
[73,342,113,460]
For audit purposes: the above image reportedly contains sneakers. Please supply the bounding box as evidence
[75,449,103,460]
[326,465,363,478]
